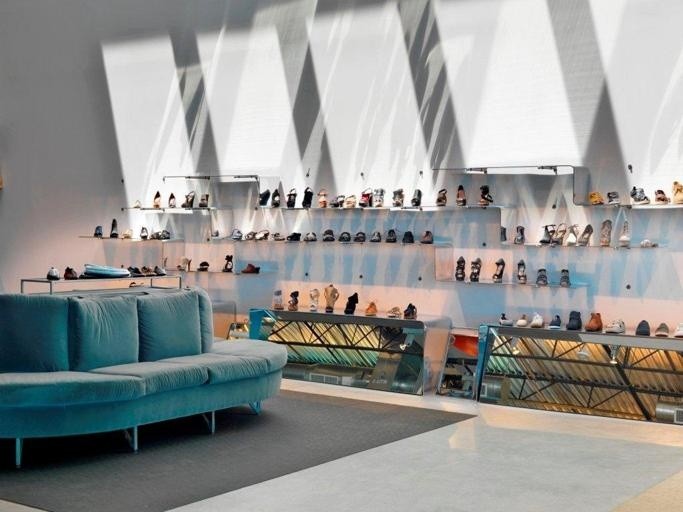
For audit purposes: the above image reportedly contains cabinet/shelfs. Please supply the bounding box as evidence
[77,164,683,291]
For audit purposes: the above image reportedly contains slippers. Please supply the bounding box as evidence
[175,255,262,274]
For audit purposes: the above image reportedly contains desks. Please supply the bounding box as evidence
[244,301,453,399]
[472,320,682,424]
[16,274,185,295]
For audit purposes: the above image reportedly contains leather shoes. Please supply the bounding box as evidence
[230,227,434,245]
[513,217,661,248]
[93,218,171,240]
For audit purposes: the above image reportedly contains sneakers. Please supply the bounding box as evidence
[587,181,683,206]
[498,311,683,339]
[128,264,167,277]
[45,266,78,280]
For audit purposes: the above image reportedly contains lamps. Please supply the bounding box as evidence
[606,344,622,365]
[508,336,521,355]
[398,334,416,351]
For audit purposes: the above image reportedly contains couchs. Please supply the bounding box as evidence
[0,288,289,469]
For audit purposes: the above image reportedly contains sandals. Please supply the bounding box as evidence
[258,184,494,210]
[133,190,210,208]
[455,254,571,287]
[272,284,419,319]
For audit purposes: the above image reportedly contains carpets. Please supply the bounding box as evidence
[0,387,478,512]
